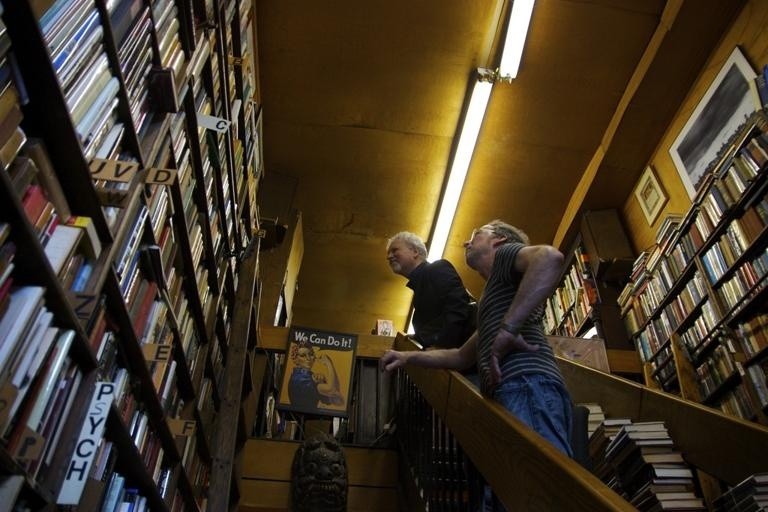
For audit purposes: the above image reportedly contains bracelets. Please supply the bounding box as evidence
[500,321,522,337]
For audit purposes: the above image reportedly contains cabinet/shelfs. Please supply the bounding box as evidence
[538,232,636,351]
[246,211,306,441]
[579,208,636,280]
[619,111,768,512]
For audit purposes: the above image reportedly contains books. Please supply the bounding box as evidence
[618,114,768,427]
[572,402,768,511]
[2,1,266,511]
[542,247,599,338]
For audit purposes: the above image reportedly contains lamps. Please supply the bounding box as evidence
[496,0,536,85]
[403,65,498,336]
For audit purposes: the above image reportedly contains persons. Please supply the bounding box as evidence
[385,231,481,387]
[377,218,576,461]
[289,343,340,407]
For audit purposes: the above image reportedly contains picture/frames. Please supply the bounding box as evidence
[631,164,671,229]
[667,42,760,204]
[340,355,393,447]
[274,324,360,418]
